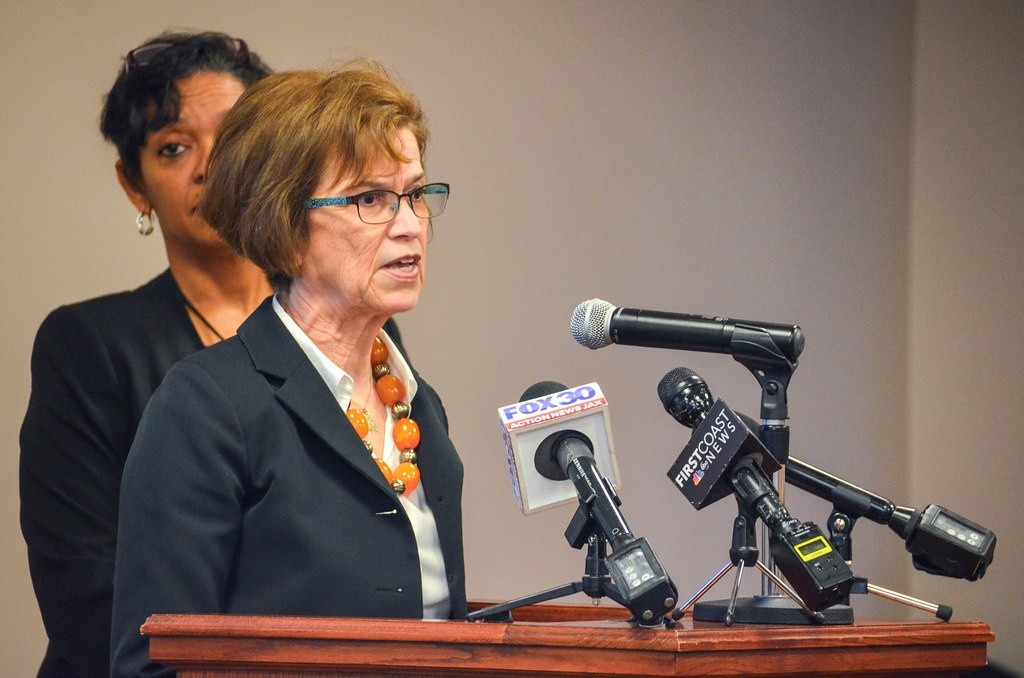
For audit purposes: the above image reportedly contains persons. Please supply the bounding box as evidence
[106,66,466,677]
[22,28,283,678]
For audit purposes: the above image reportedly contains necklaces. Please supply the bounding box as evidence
[348,335,423,497]
[184,297,226,343]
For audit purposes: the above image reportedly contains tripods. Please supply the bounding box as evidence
[827,504,954,620]
[669,510,826,629]
[463,497,675,631]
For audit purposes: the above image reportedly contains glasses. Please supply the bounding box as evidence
[302,182,451,223]
[125,41,175,76]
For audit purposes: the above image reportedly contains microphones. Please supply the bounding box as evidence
[498,298,996,627]
